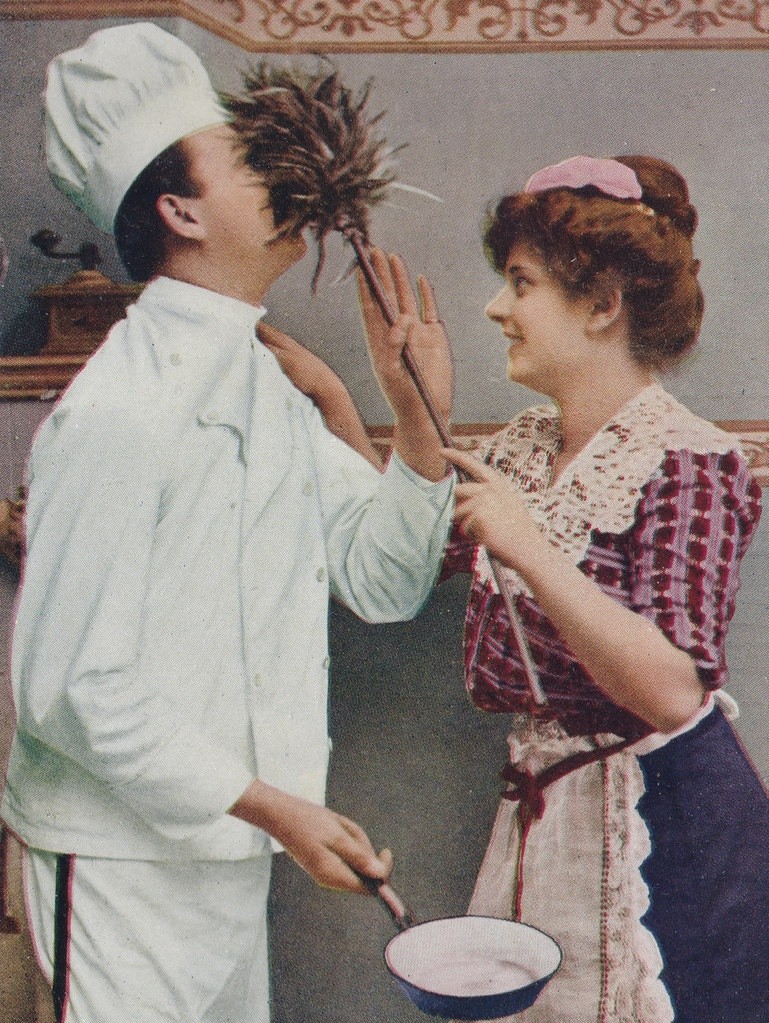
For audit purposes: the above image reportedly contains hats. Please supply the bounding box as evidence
[44,24,238,236]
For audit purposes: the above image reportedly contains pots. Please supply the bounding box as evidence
[359,877,562,1020]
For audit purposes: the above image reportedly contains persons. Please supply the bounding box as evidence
[254,150,768,1022]
[5,17,458,1020]
